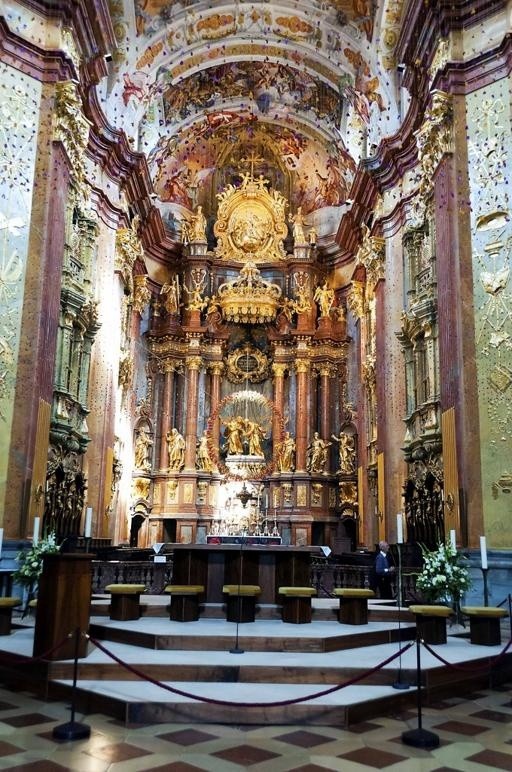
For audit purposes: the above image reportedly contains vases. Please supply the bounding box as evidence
[22,581,38,620]
[448,589,467,629]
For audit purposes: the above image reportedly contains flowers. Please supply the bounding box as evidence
[14,529,63,596]
[416,539,473,608]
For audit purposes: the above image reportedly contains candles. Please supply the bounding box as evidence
[448,529,458,555]
[395,512,405,545]
[83,506,95,540]
[0,527,5,557]
[257,494,277,507]
[32,515,41,547]
[478,535,489,570]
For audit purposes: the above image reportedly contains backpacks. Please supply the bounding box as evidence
[368,557,386,588]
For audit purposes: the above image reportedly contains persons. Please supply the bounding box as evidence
[158,271,336,320]
[190,206,209,241]
[290,206,310,245]
[376,540,397,599]
[134,414,360,476]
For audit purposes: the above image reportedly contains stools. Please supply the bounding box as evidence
[222,584,261,623]
[104,582,147,622]
[462,606,507,645]
[1,597,24,637]
[334,587,374,625]
[278,586,315,624]
[409,605,454,645]
[165,585,204,622]
